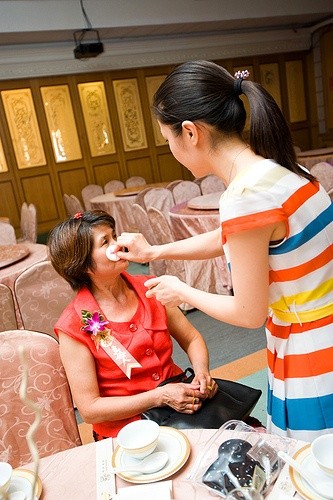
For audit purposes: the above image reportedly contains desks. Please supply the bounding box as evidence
[12,430,312,500]
[88,181,187,263]
[295,147,333,171]
[0,243,52,329]
[169,190,232,309]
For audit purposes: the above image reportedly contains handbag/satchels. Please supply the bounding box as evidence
[185,420,292,500]
[141,367,262,431]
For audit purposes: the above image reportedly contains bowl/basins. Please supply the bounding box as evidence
[117,418,160,459]
[311,432,333,475]
[0,462,13,495]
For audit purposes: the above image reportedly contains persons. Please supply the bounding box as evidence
[48,209,266,443]
[113,61,333,440]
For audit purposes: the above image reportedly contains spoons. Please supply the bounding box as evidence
[277,450,333,500]
[107,452,168,476]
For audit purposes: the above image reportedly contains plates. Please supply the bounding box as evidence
[0,469,42,500]
[111,427,191,484]
[289,443,333,500]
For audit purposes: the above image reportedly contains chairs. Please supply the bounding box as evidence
[0,147,333,500]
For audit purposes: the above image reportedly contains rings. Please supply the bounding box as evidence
[206,386,213,391]
[194,398,197,404]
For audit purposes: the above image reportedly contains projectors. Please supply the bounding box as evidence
[73,43,104,59]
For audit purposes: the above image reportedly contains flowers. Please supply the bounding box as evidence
[80,309,111,338]
